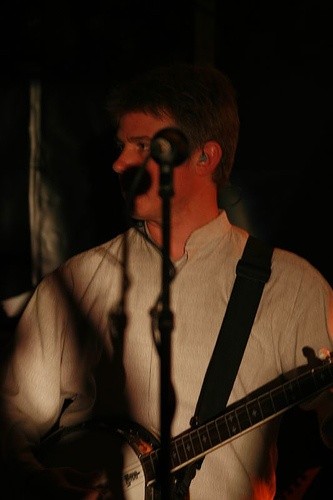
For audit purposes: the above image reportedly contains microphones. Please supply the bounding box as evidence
[148,126,191,167]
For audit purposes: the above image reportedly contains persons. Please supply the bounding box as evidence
[0,61,333,500]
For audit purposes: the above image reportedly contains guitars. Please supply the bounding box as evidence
[37,359,333,500]
